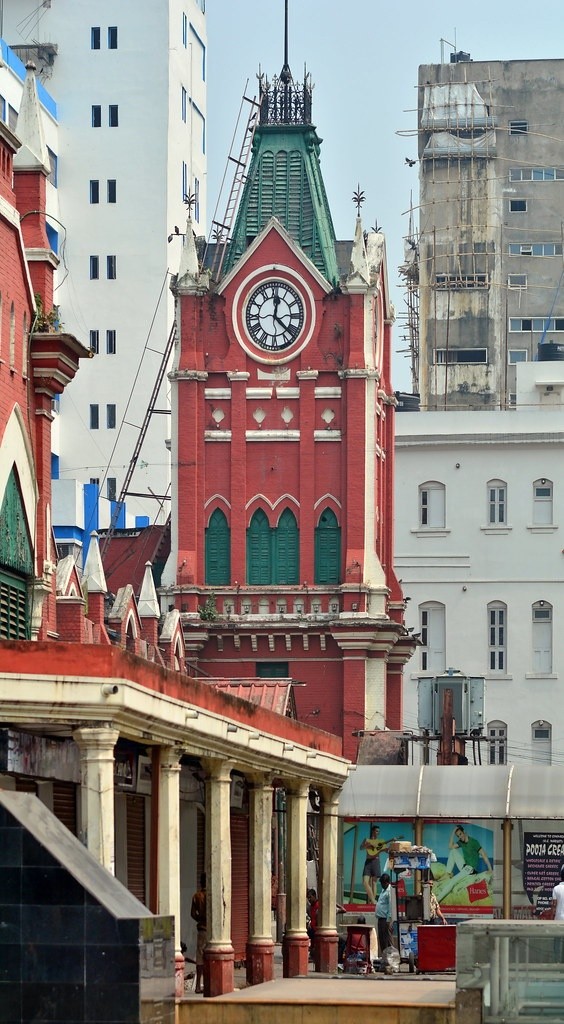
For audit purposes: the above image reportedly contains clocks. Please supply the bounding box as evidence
[242,277,307,355]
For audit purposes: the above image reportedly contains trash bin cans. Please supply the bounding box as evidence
[416,925,457,975]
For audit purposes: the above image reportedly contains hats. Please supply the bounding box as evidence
[426,879,434,886]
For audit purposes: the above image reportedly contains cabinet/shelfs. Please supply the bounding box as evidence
[389,850,435,971]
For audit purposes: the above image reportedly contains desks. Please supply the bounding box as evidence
[415,924,456,975]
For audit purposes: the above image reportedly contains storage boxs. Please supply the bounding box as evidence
[389,841,411,852]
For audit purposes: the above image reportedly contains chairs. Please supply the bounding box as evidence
[343,926,372,975]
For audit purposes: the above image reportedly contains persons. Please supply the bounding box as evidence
[360,827,393,905]
[179,874,449,995]
[437,823,496,902]
[550,867,564,964]
